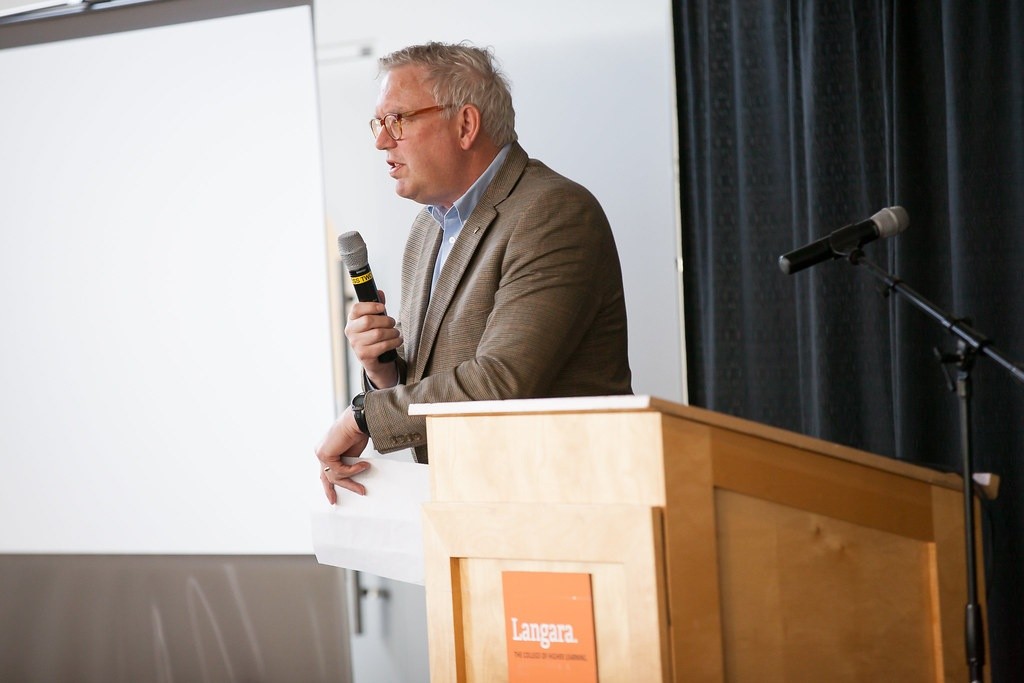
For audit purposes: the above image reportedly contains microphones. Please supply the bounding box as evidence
[337,231,398,364]
[779,205,909,274]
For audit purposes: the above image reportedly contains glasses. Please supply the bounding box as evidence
[370,103,458,141]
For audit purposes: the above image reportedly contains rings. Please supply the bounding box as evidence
[324,466,330,471]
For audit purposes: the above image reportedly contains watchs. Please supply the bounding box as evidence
[351,391,372,437]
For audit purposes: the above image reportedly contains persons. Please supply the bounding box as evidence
[313,43,637,505]
